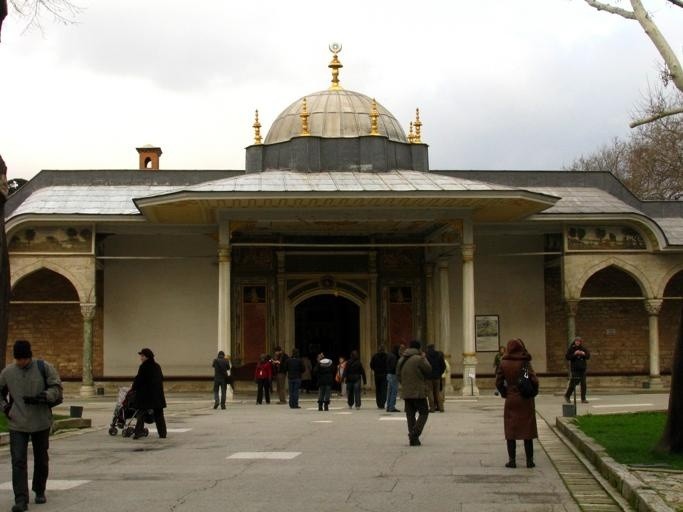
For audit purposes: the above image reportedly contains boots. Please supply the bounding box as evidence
[506,448,516,467]
[318,400,329,410]
[525,448,535,468]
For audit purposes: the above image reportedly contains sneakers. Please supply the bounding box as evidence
[387,408,401,412]
[581,399,588,403]
[408,430,421,446]
[214,401,226,409]
[564,394,571,403]
[134,431,144,439]
[12,500,28,511]
[35,492,46,503]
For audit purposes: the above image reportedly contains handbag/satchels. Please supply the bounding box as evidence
[520,369,539,399]
[226,376,232,384]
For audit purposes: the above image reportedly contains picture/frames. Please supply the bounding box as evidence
[475,314,500,352]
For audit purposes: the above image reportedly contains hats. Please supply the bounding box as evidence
[13,340,33,359]
[138,348,155,358]
[409,341,420,350]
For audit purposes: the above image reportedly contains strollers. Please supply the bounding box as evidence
[109,388,156,438]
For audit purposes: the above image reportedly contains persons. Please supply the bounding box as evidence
[492,346,506,396]
[131,347,168,439]
[563,336,590,404]
[495,336,539,469]
[254,342,447,413]
[0,340,63,512]
[395,339,433,447]
[212,350,231,410]
